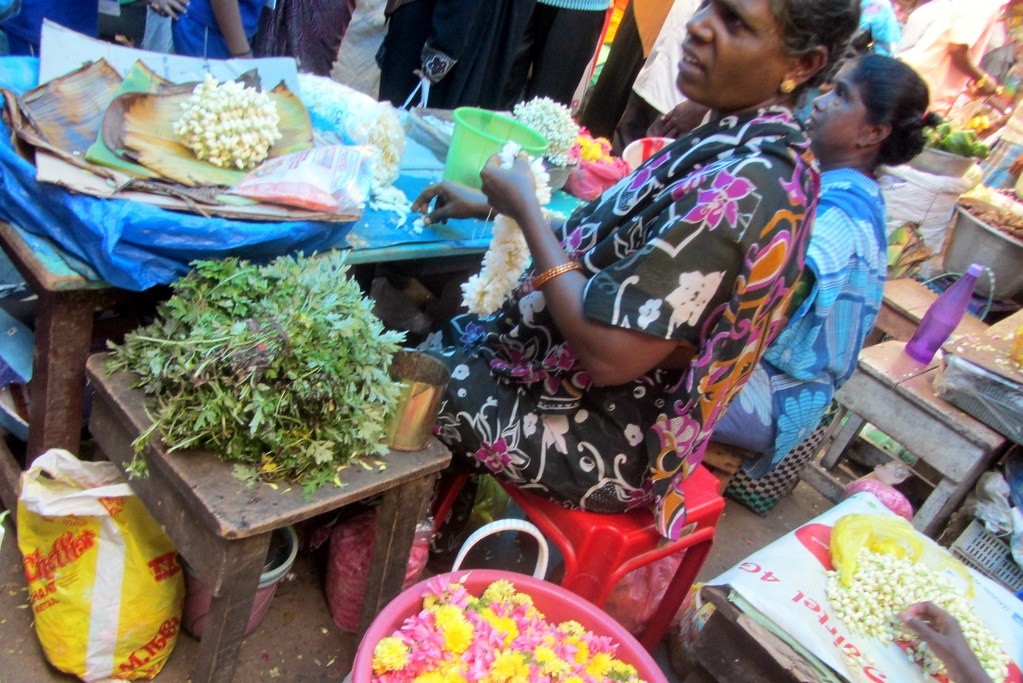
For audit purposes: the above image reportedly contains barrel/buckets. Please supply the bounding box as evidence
[442,106,550,191]
[343,569,671,683]
[378,348,451,452]
[183,526,298,639]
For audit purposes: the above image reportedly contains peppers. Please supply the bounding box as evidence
[924,119,989,158]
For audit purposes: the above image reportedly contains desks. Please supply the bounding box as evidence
[676,490,1023,683]
[0,106,591,525]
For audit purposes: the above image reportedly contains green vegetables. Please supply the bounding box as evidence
[106,248,408,495]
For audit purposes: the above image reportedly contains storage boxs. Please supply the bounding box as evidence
[949,517,1023,596]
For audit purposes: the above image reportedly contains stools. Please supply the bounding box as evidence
[85,352,453,683]
[432,458,725,656]
[863,278,989,348]
[799,335,1008,539]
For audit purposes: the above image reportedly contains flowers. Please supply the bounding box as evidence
[370,571,648,683]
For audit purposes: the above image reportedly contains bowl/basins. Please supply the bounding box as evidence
[908,146,977,180]
[621,136,675,172]
[942,204,1023,301]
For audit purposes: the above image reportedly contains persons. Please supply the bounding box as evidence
[898,601,996,683]
[707,55,929,479]
[375,0,479,110]
[0,1,362,79]
[947,0,1023,142]
[890,0,996,120]
[481,1,612,117]
[417,0,860,514]
[609,0,710,158]
[792,0,902,125]
[580,1,675,142]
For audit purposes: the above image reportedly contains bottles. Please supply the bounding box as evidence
[905,264,983,365]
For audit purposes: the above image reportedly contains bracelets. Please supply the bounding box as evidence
[532,261,584,290]
[976,73,989,90]
